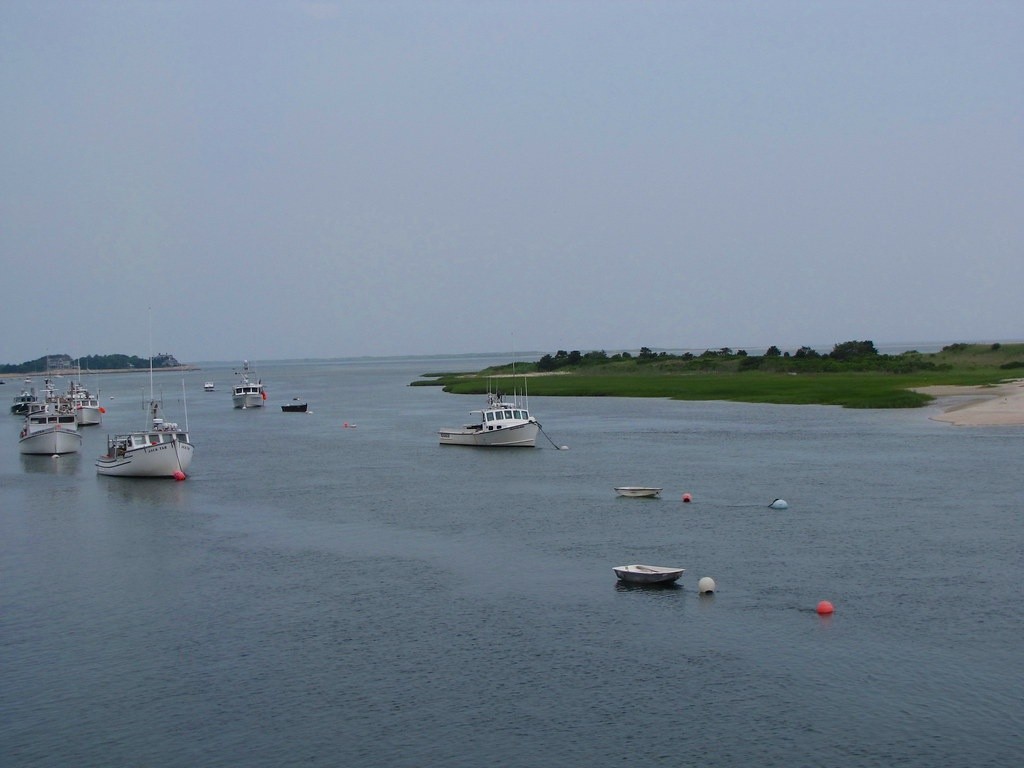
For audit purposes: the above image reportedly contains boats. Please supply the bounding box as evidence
[12,377,39,414]
[614,487,663,497]
[437,330,539,446]
[613,564,685,583]
[232,359,266,409]
[96,304,193,479]
[204,381,214,392]
[281,403,307,412]
[17,378,82,455]
[65,380,102,427]
